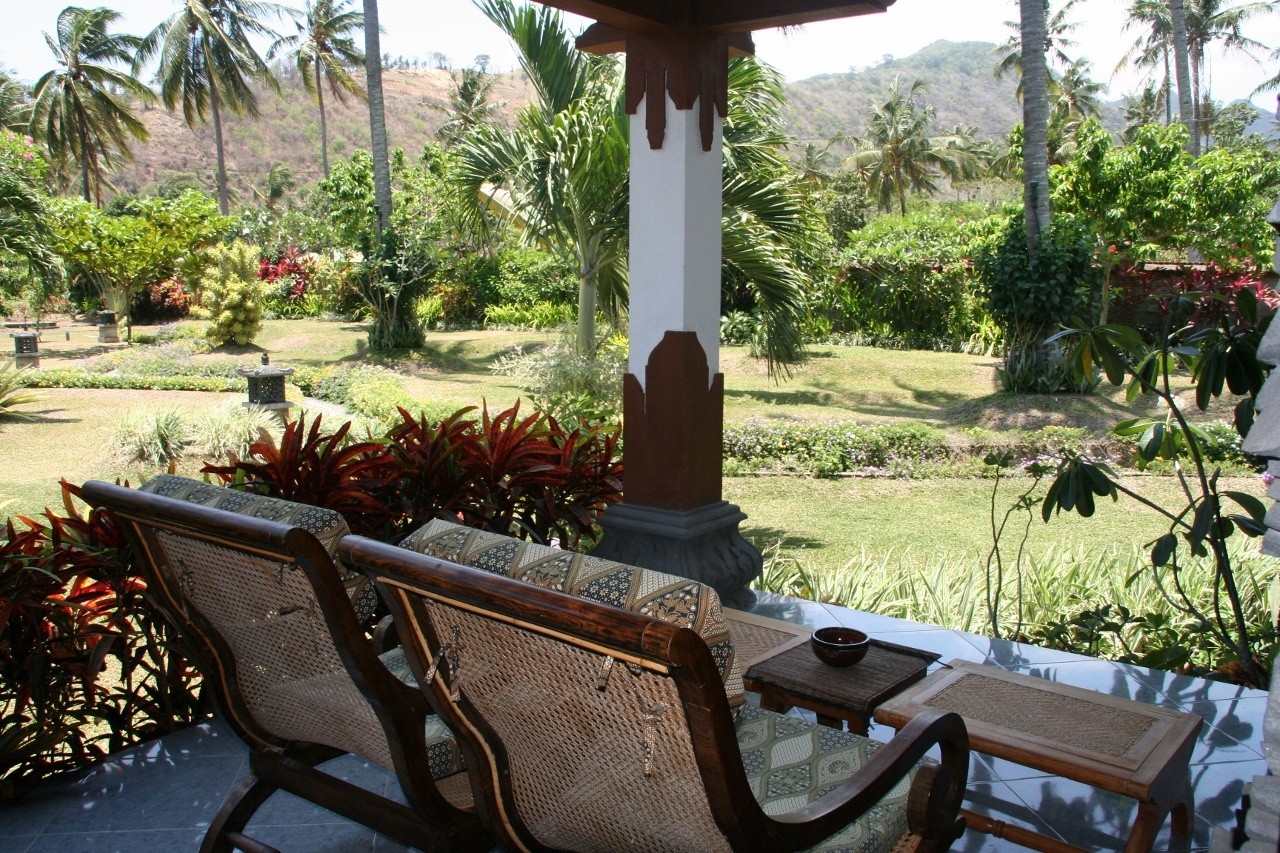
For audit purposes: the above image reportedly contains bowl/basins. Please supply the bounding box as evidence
[811,626,870,669]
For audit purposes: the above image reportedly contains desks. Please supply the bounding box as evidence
[722,606,812,707]
[873,660,1203,853]
[742,629,942,736]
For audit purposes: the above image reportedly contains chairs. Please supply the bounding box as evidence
[339,517,973,853]
[80,471,500,853]
